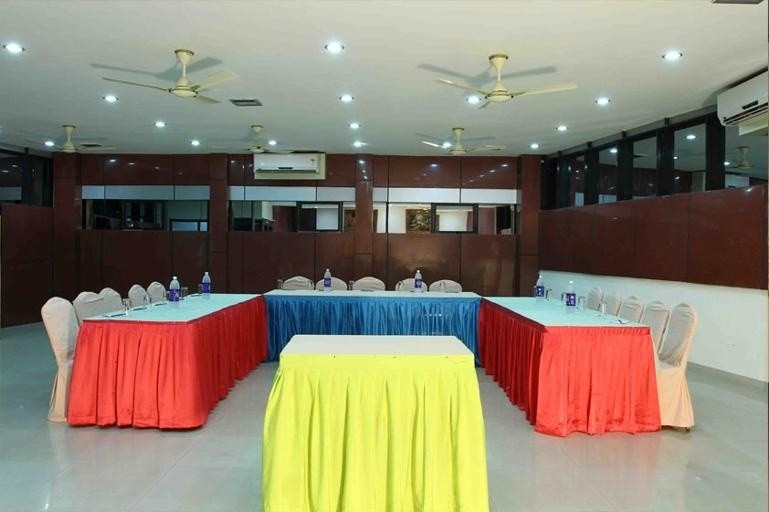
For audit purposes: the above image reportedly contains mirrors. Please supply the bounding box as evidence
[79,179,216,233]
[369,184,523,239]
[0,142,55,208]
[532,107,769,215]
[225,182,358,236]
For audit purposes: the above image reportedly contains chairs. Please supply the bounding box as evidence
[38,278,170,425]
[585,283,698,436]
[281,269,468,295]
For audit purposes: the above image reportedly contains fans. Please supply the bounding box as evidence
[101,47,236,108]
[433,51,579,109]
[423,125,504,156]
[22,123,119,158]
[720,144,757,171]
[214,123,294,157]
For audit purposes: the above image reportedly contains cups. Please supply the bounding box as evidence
[577,296,586,313]
[560,293,566,302]
[199,284,203,295]
[277,279,284,289]
[546,288,554,302]
[597,303,608,318]
[122,298,131,315]
[143,295,153,312]
[439,282,446,294]
[348,281,355,291]
[180,287,188,301]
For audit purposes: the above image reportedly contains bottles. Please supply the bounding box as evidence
[414,270,422,294]
[563,281,577,313]
[536,274,545,303]
[169,276,180,303]
[324,268,331,292]
[202,271,211,300]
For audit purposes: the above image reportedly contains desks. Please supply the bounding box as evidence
[67,291,270,435]
[262,289,480,371]
[477,294,662,437]
[262,329,493,511]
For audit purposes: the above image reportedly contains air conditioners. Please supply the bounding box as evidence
[715,65,768,131]
[250,151,321,176]
